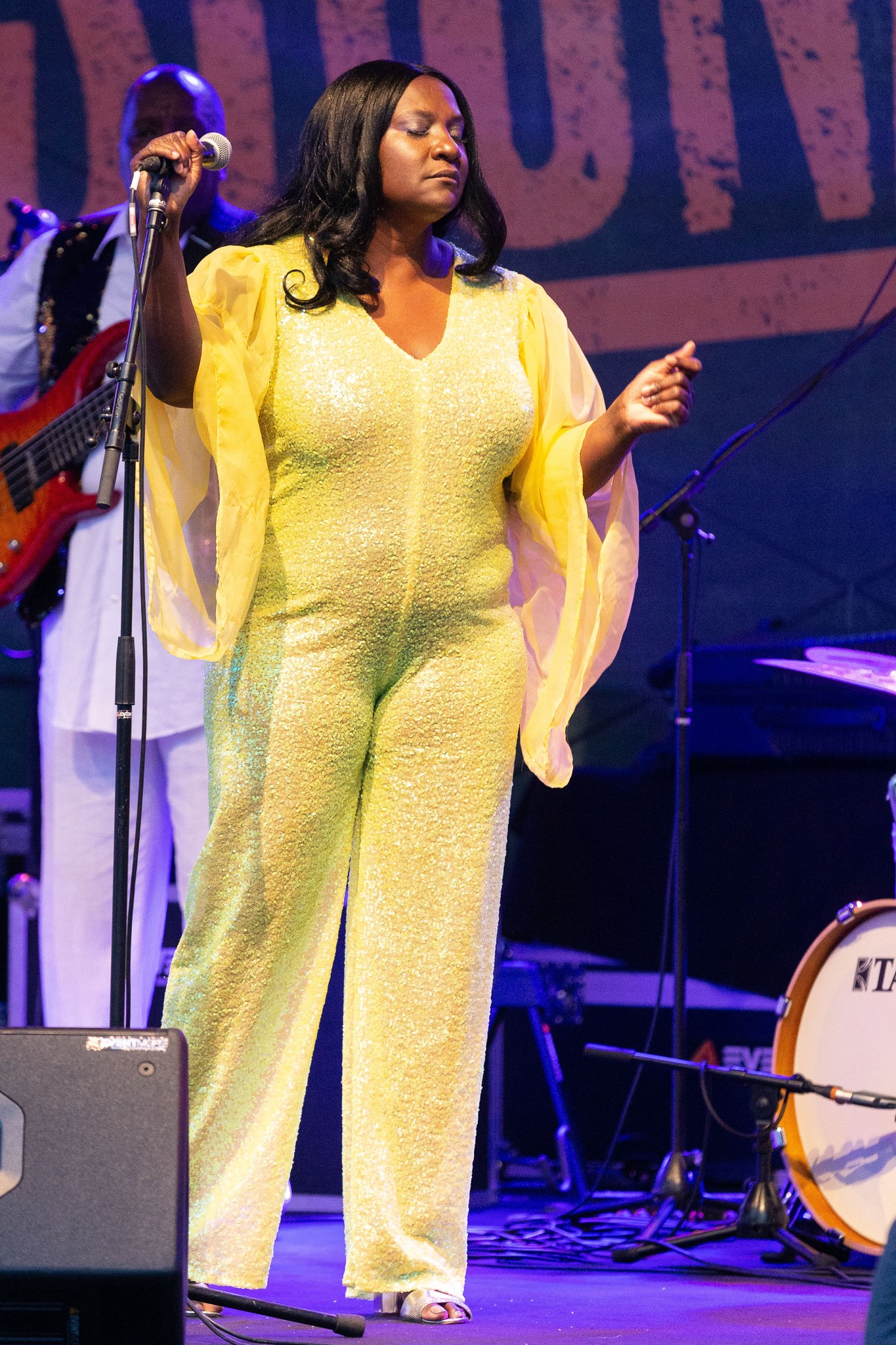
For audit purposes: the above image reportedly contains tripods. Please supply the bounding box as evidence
[578,1045,895,1290]
[563,295,895,1240]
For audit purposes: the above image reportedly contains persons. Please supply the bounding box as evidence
[0,61,274,1040]
[125,51,701,1322]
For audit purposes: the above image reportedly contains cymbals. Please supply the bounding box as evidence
[753,646,896,696]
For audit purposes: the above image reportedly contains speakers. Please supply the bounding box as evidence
[0,1026,187,1341]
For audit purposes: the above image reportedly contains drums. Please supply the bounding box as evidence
[773,897,895,1257]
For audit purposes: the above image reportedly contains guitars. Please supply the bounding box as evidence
[1,297,147,629]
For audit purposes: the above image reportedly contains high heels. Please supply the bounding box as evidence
[372,1290,473,1325]
[185,1282,226,1317]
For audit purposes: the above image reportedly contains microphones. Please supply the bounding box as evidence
[137,132,234,173]
[6,196,58,236]
